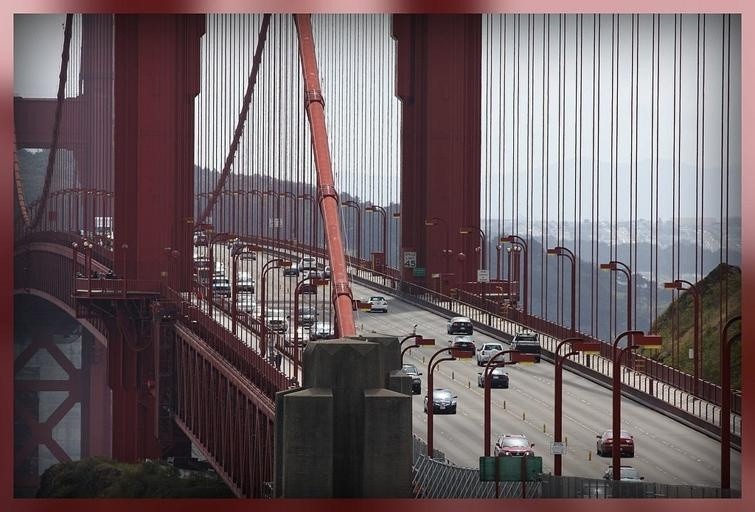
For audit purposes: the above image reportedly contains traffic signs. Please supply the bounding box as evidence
[402,251,417,270]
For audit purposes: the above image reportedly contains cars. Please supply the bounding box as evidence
[475,367,512,389]
[602,465,646,481]
[446,335,477,357]
[70,213,337,346]
[445,316,475,336]
[592,427,636,458]
[421,385,460,415]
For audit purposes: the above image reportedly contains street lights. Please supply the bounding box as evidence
[397,331,437,365]
[294,274,328,380]
[611,329,667,480]
[188,188,399,312]
[258,256,292,357]
[21,186,112,239]
[423,213,451,272]
[425,344,472,459]
[663,277,703,401]
[482,348,539,457]
[544,245,578,339]
[596,258,635,374]
[552,337,603,476]
[439,218,531,318]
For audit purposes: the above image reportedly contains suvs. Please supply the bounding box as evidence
[475,342,506,367]
[403,363,424,398]
[493,433,537,458]
[505,333,543,365]
